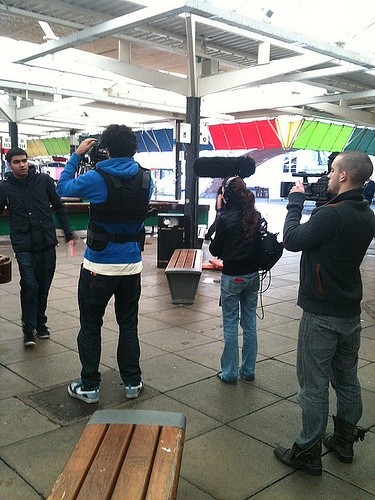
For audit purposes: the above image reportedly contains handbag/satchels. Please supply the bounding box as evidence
[244,217,284,273]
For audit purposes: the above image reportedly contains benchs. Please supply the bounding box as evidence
[47,409,188,500]
[163,249,203,306]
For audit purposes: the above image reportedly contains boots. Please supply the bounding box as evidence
[273,437,323,477]
[322,414,367,463]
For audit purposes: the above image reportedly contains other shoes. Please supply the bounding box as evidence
[238,368,256,381]
[216,371,238,386]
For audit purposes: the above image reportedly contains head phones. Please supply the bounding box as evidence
[222,175,239,201]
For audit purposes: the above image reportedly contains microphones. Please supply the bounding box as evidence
[193,154,256,180]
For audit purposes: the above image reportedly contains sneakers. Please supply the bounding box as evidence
[23,333,37,348]
[124,380,144,399]
[36,324,51,339]
[67,381,100,404]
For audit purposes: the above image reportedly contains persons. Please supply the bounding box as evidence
[56,124,154,403]
[274,150,375,476]
[0,148,77,348]
[209,175,261,385]
